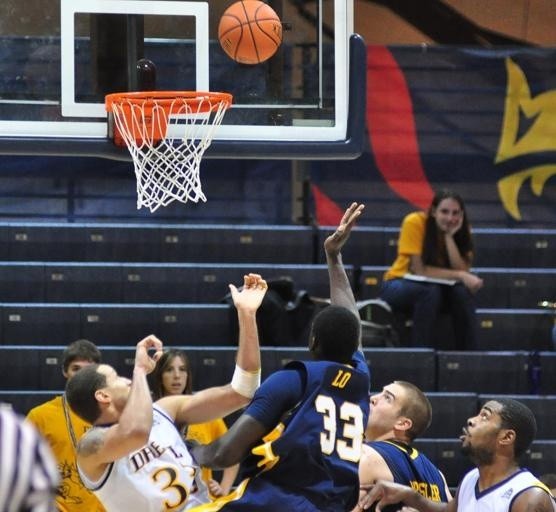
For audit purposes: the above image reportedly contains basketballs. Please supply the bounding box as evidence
[219,0,282,63]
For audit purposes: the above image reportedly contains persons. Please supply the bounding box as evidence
[1,402,62,511]
[145,349,241,498]
[192,203,370,512]
[353,380,454,512]
[379,189,484,349]
[65,273,268,512]
[27,339,108,512]
[359,397,555,512]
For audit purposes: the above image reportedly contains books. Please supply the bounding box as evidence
[404,272,462,286]
